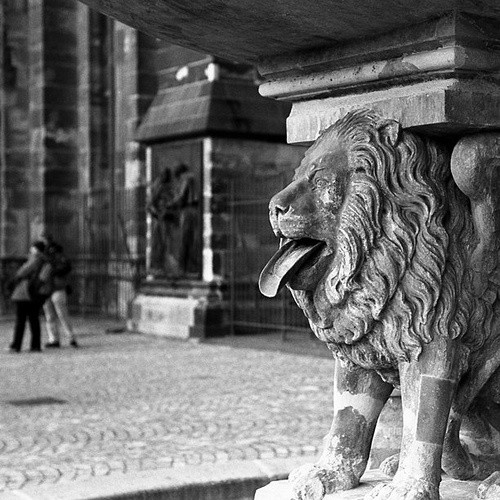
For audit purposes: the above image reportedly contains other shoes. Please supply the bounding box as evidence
[70,340,77,347]
[45,340,60,347]
[11,342,20,352]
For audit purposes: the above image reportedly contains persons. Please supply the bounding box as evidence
[37,230,78,348]
[9,241,51,352]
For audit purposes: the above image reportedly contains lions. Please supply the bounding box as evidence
[259,107,500,500]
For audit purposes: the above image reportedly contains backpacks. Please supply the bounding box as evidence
[25,253,54,304]
